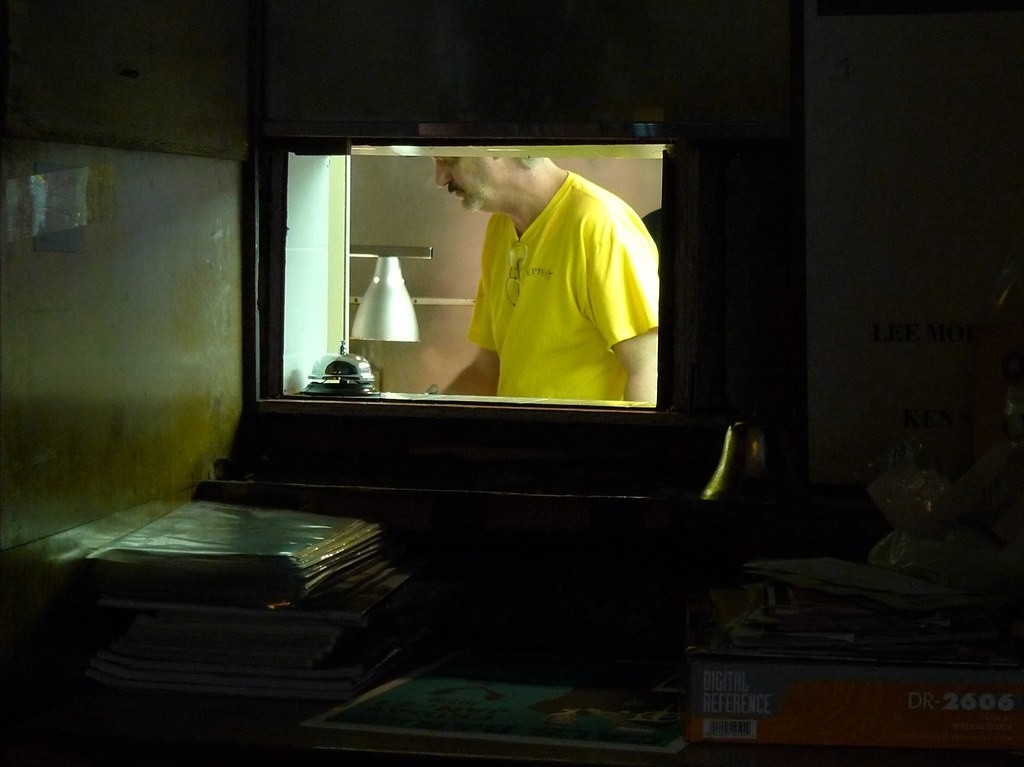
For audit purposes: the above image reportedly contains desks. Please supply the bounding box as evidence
[0,641,1024,767]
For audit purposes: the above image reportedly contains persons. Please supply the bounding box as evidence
[431,156,659,401]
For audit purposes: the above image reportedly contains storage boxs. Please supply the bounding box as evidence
[683,654,1024,749]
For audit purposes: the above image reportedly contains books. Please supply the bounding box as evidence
[683,557,1023,753]
[87,498,412,700]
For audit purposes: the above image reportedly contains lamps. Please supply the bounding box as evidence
[700,420,773,505]
[350,256,418,343]
